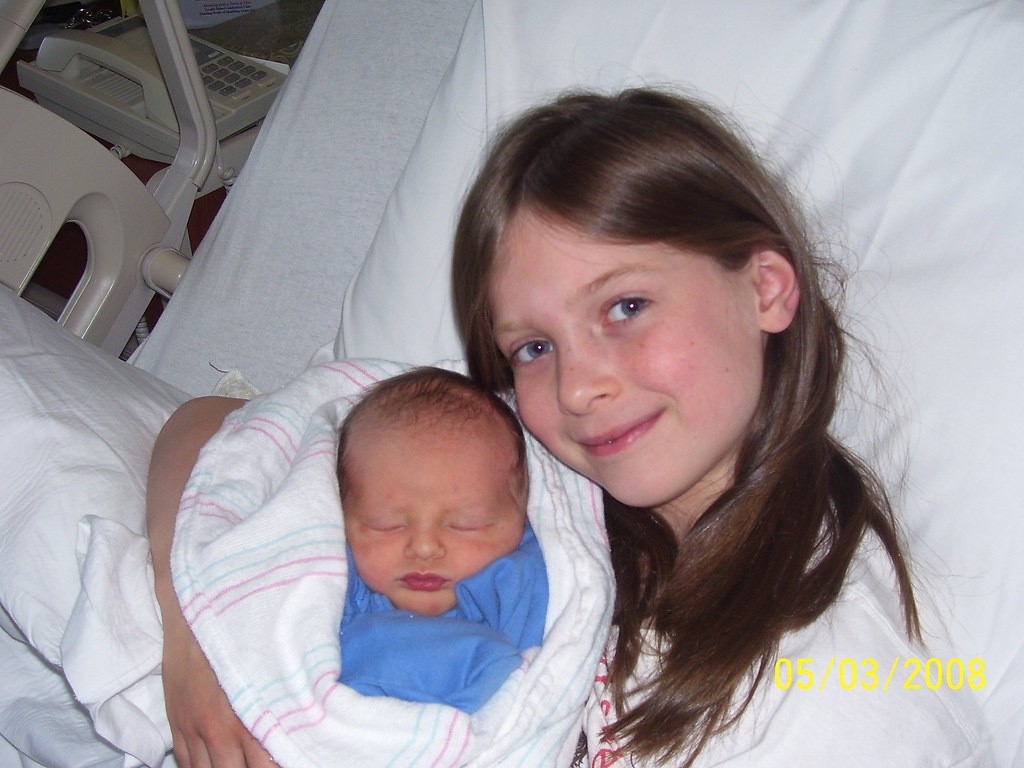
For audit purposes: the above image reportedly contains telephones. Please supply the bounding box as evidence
[14,11,290,166]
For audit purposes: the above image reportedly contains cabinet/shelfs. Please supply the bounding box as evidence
[0,0,323,342]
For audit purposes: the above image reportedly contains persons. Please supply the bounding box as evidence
[171,357,615,766]
[146,83,989,768]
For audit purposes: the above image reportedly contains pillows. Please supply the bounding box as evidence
[302,0,1024,768]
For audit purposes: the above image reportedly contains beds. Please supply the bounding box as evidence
[0,0,487,767]
